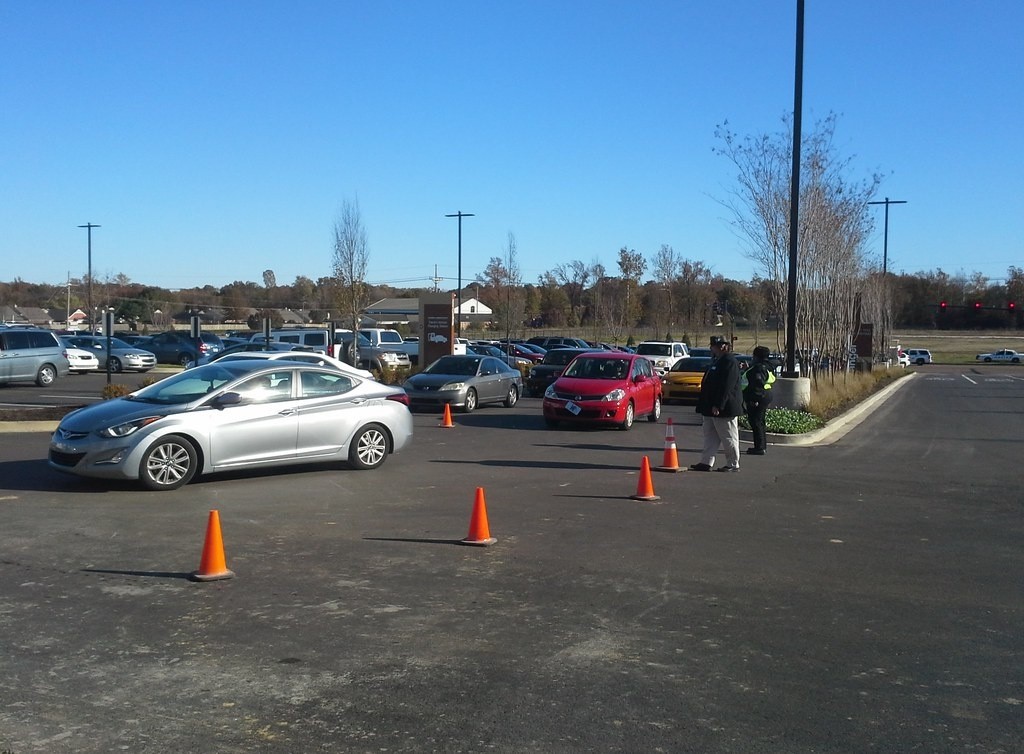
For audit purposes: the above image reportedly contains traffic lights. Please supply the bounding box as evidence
[940,301,946,311]
[1008,301,1014,312]
[975,300,980,309]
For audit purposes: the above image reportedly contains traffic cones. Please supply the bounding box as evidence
[632,456,661,502]
[461,486,500,545]
[190,509,235,582]
[439,403,455,428]
[655,418,688,472]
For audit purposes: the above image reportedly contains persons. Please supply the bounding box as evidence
[741,346,775,455]
[690,335,744,473]
[612,362,626,379]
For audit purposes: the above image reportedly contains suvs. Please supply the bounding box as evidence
[467,345,533,378]
[905,349,931,366]
[133,330,223,366]
[637,340,691,379]
[0,320,69,387]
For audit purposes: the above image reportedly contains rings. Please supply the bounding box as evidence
[714,412,715,414]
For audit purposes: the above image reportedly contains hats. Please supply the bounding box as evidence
[708,335,733,346]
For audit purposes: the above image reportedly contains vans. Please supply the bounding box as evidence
[249,329,374,363]
[360,329,411,371]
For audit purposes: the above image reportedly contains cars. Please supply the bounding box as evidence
[70,336,157,373]
[401,354,524,413]
[976,348,1024,363]
[527,348,604,398]
[454,338,471,356]
[734,353,807,378]
[473,338,547,364]
[899,352,910,367]
[186,342,319,366]
[221,337,246,349]
[60,337,99,375]
[210,351,376,377]
[48,359,418,490]
[587,342,634,354]
[51,328,139,345]
[687,348,717,357]
[402,336,419,344]
[543,353,662,430]
[660,357,717,404]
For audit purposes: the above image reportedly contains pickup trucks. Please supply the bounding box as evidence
[528,336,587,348]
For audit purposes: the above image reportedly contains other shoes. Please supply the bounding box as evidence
[690,463,712,472]
[717,466,740,472]
[747,448,768,455]
